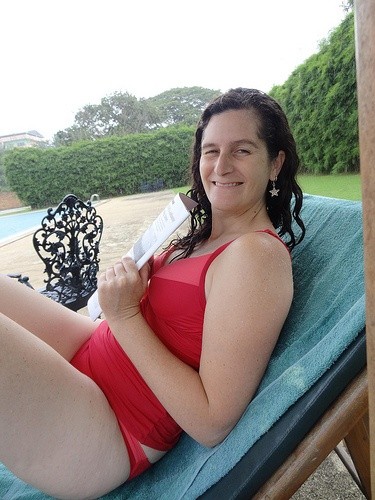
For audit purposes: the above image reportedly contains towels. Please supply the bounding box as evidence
[0,184,372,496]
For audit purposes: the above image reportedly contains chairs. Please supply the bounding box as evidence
[1,192,375,500]
[6,195,103,312]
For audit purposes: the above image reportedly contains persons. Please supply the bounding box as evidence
[0,87,305,499]
